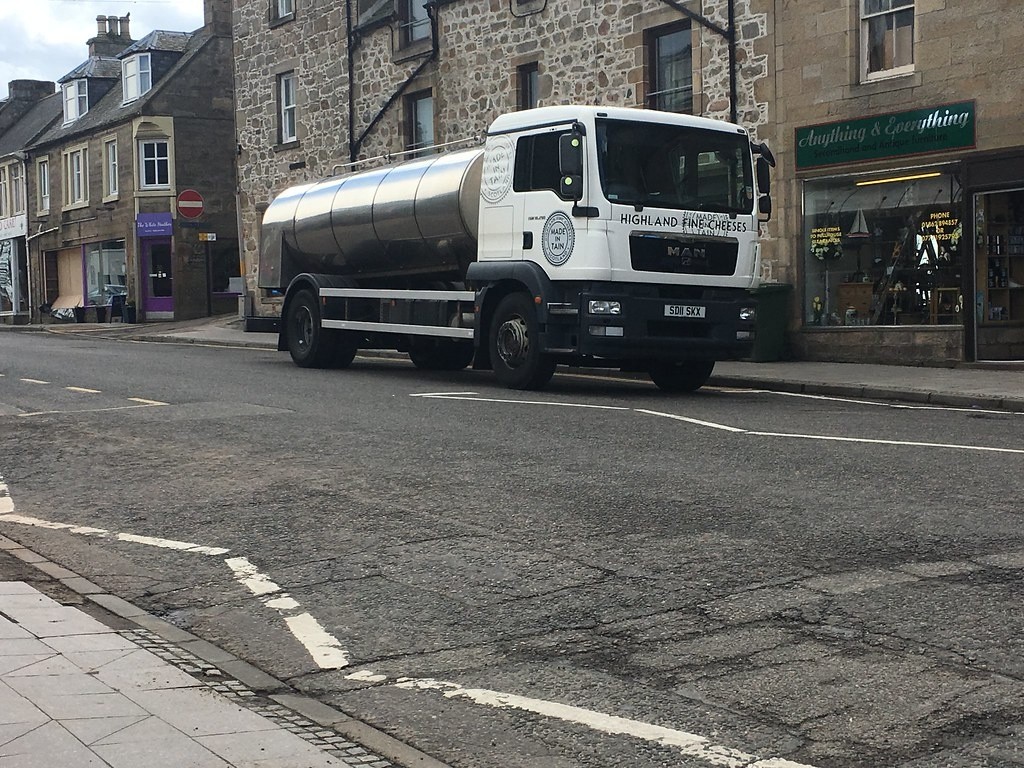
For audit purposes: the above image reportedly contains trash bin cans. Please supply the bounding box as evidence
[748,282,794,363]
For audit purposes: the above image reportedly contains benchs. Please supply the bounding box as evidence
[72,304,113,323]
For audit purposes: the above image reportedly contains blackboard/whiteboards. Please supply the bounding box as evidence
[111,295,126,317]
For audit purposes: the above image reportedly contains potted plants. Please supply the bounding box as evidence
[122,298,135,324]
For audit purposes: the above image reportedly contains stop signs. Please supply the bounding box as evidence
[177,190,205,220]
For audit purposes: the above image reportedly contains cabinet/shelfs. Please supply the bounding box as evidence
[975,208,1024,321]
[874,266,961,324]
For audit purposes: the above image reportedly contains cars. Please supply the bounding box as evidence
[87,284,127,304]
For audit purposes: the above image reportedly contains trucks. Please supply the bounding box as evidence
[243,103,776,396]
